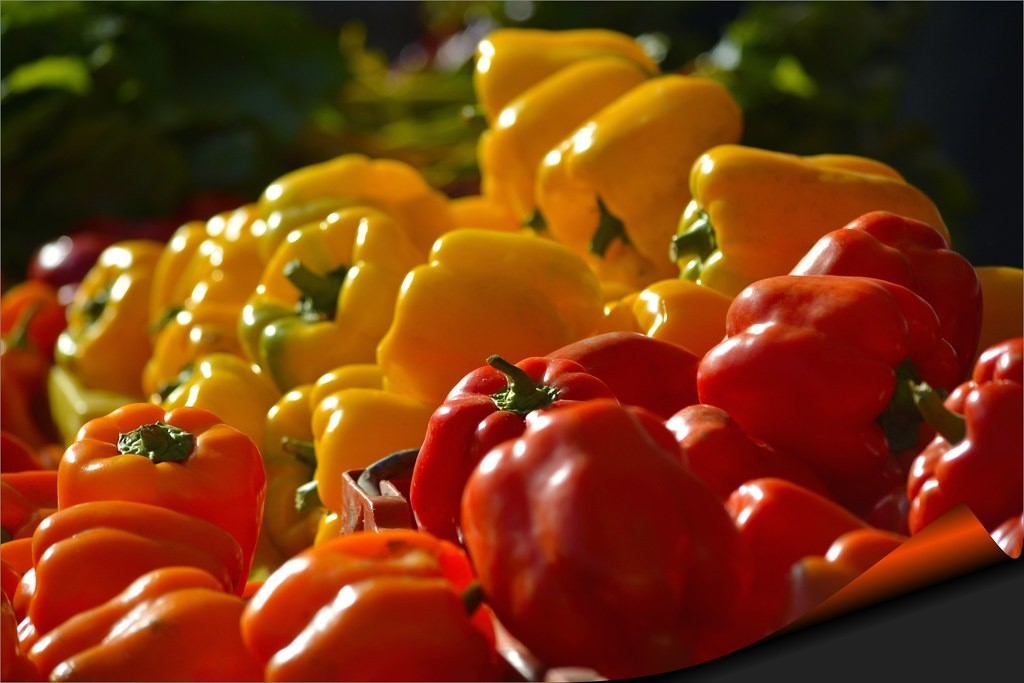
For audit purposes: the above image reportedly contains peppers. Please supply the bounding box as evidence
[0,1,1024,683]
